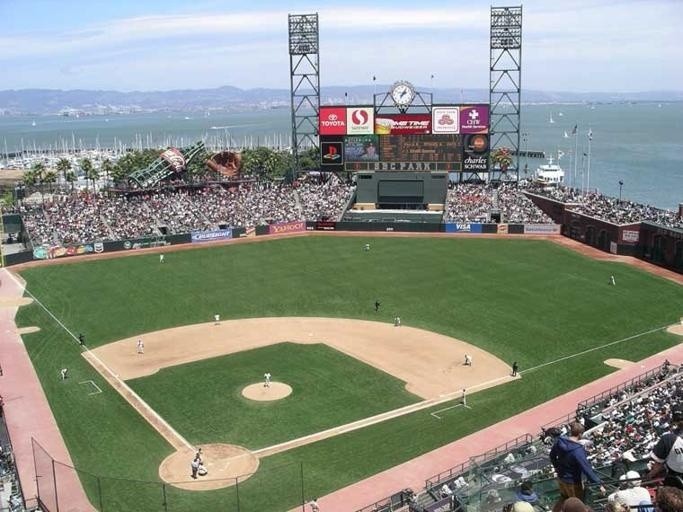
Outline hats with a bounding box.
[620,471,642,484]
[511,501,534,512]
[561,497,588,512]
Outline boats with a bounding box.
[530,152,565,187]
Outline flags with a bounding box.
[557,124,592,160]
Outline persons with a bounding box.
[79,333,86,346]
[376,299,382,312]
[607,271,616,285]
[159,253,166,263]
[394,316,400,326]
[136,339,144,354]
[214,313,220,325]
[360,142,379,161]
